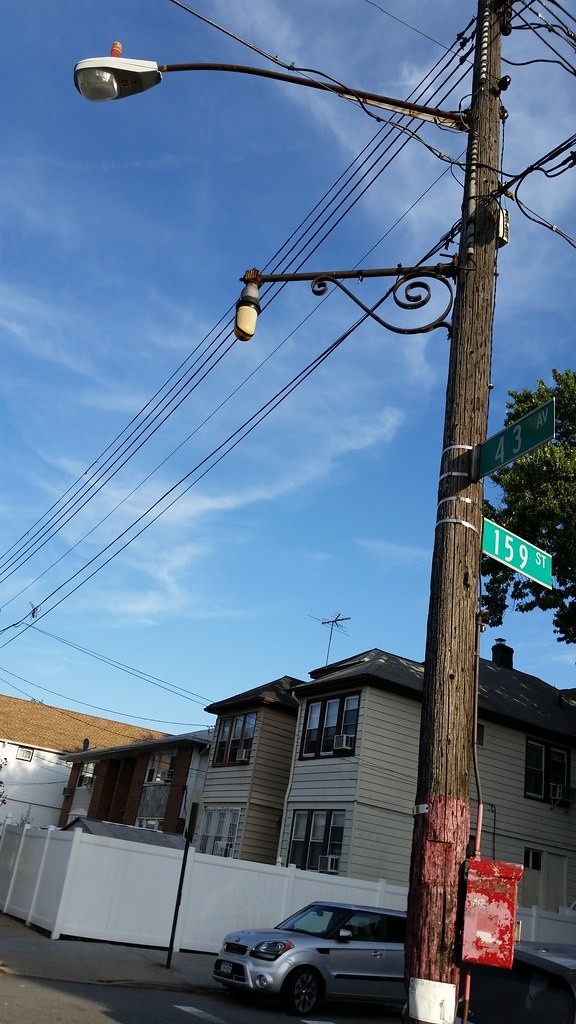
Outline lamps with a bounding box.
[318,855,340,872]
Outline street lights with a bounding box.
[72,37,507,1024]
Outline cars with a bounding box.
[211,898,411,1020]
[459,938,576,1024]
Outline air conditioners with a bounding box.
[236,749,251,760]
[549,783,576,802]
[332,734,354,749]
